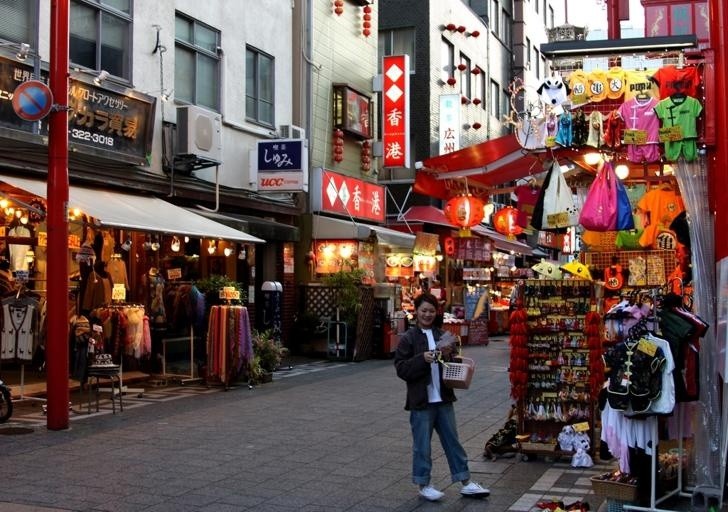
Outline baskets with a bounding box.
[605,496,632,512]
[590,473,639,502]
[442,355,475,389]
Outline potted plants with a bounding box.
[253,328,288,385]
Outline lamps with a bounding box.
[121,236,249,260]
[0,40,32,63]
[146,87,174,102]
[77,67,109,85]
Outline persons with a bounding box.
[393,293,490,501]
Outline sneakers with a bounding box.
[418,485,446,502]
[461,481,491,498]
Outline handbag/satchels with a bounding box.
[529,159,635,232]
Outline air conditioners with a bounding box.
[174,102,224,165]
[280,124,306,142]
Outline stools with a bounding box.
[76,365,124,416]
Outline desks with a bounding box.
[489,307,510,336]
[440,320,470,347]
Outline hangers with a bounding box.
[9,283,29,310]
[599,284,688,348]
